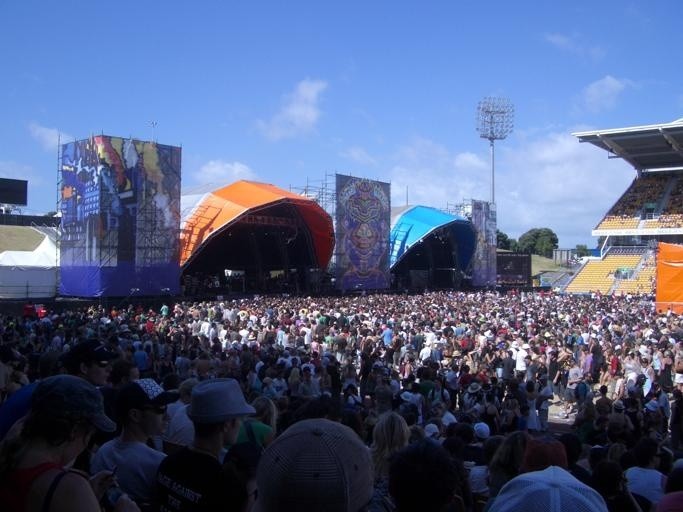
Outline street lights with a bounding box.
[475,94,516,206]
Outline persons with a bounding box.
[187,269,288,286]
[605,174,683,228]
[635,244,655,286]
[1,286,682,512]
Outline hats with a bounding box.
[34,374,116,432]
[426,424,439,436]
[186,378,256,415]
[507,350,513,355]
[525,355,531,359]
[118,378,182,410]
[600,385,607,390]
[645,400,658,409]
[401,391,412,401]
[468,382,481,393]
[540,375,547,379]
[474,423,490,438]
[613,399,625,408]
[524,440,568,469]
[639,374,648,380]
[251,418,373,512]
[70,342,119,367]
